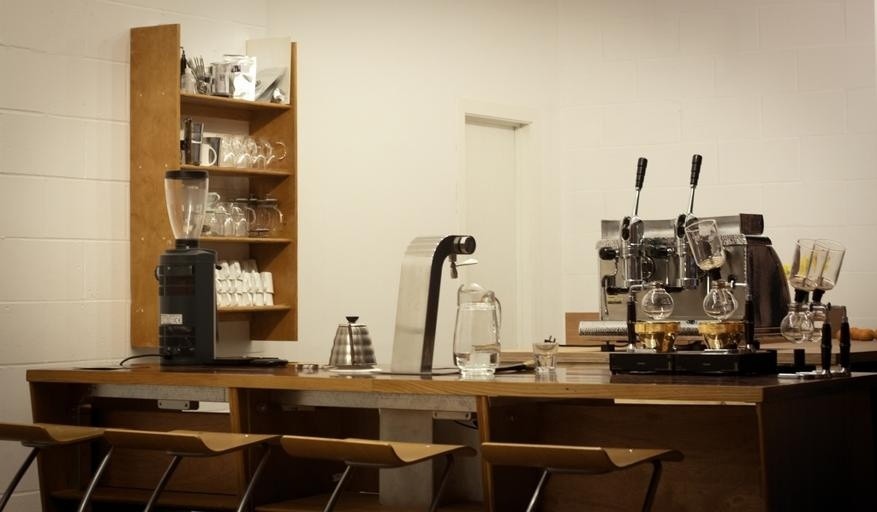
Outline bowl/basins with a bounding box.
[694,319,747,349]
[632,320,681,354]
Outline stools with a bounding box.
[104,428,283,512]
[281,436,478,512]
[2,420,114,512]
[480,441,683,512]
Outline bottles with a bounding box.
[785,236,829,292]
[805,238,847,292]
[451,281,504,376]
[682,217,726,273]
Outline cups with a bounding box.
[179,115,287,306]
[531,341,559,374]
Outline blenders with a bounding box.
[154,166,219,368]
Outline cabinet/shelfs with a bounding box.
[126,23,301,351]
